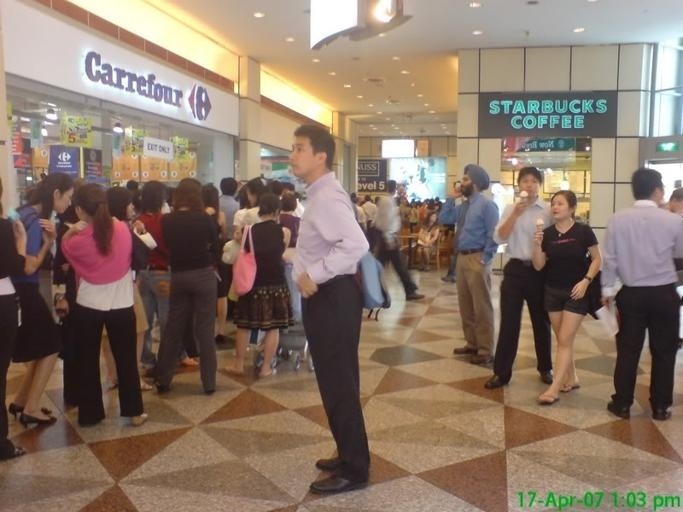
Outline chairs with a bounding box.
[440,232,453,267]
[428,234,442,269]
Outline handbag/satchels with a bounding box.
[417,232,432,247]
[566,271,607,317]
[232,248,258,297]
[222,239,242,266]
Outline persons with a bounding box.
[532,190,602,406]
[0,171,305,458]
[287,124,370,495]
[438,163,499,365]
[647,188,682,354]
[484,167,554,389]
[349,180,468,302]
[599,169,682,420]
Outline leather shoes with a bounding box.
[155,383,174,394]
[80,419,102,426]
[315,456,344,474]
[538,370,554,385]
[204,389,216,395]
[310,471,368,495]
[484,374,511,390]
[9,447,26,458]
[214,333,236,346]
[453,347,477,355]
[469,354,495,365]
[406,290,425,302]
[128,412,149,426]
[652,407,673,420]
[607,401,631,419]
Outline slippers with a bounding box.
[535,393,559,405]
[220,364,245,376]
[559,381,581,393]
[258,368,277,380]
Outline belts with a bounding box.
[510,258,537,269]
[460,246,485,254]
[315,274,355,288]
[139,264,169,271]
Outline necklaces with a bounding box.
[556,220,573,237]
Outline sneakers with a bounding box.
[440,275,456,284]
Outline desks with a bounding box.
[395,235,418,270]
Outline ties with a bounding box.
[452,201,471,252]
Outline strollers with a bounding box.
[254,263,323,373]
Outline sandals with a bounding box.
[138,361,158,372]
[107,375,154,392]
[174,358,199,373]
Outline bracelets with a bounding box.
[584,276,592,283]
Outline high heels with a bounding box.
[8,403,52,419]
[20,411,57,429]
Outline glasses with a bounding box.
[61,190,74,203]
